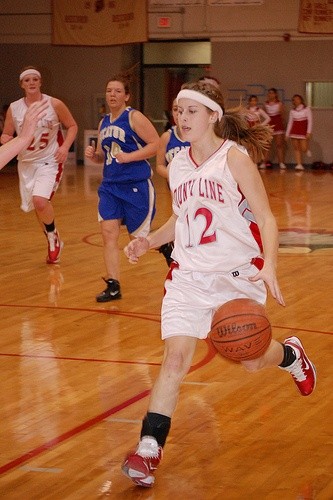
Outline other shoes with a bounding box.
[260,163,266,168]
[296,164,305,170]
[280,163,287,168]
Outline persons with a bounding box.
[154,98,191,188]
[264,88,286,169]
[200,76,219,88]
[118,80,317,488]
[85,78,174,302]
[283,93,313,169]
[0,66,77,264]
[0,98,49,169]
[244,95,271,168]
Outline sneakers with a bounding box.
[96,276,121,302]
[157,242,175,266]
[41,228,64,263]
[120,437,163,486]
[277,336,316,395]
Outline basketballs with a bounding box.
[212,299,272,361]
